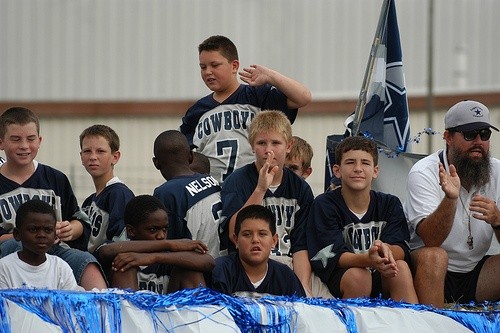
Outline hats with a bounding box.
[444,100,499,131]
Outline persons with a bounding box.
[93,195,216,290]
[0,106,110,289]
[79,125,136,252]
[0,200,82,291]
[179,35,311,183]
[190,152,210,175]
[209,205,306,297]
[153,131,227,261]
[307,136,419,304]
[404,100,500,307]
[220,110,314,296]
[285,136,313,180]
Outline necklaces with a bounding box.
[459,193,473,250]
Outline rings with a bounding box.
[445,182,447,185]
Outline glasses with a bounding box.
[454,128,493,141]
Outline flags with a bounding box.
[346,0,410,153]
